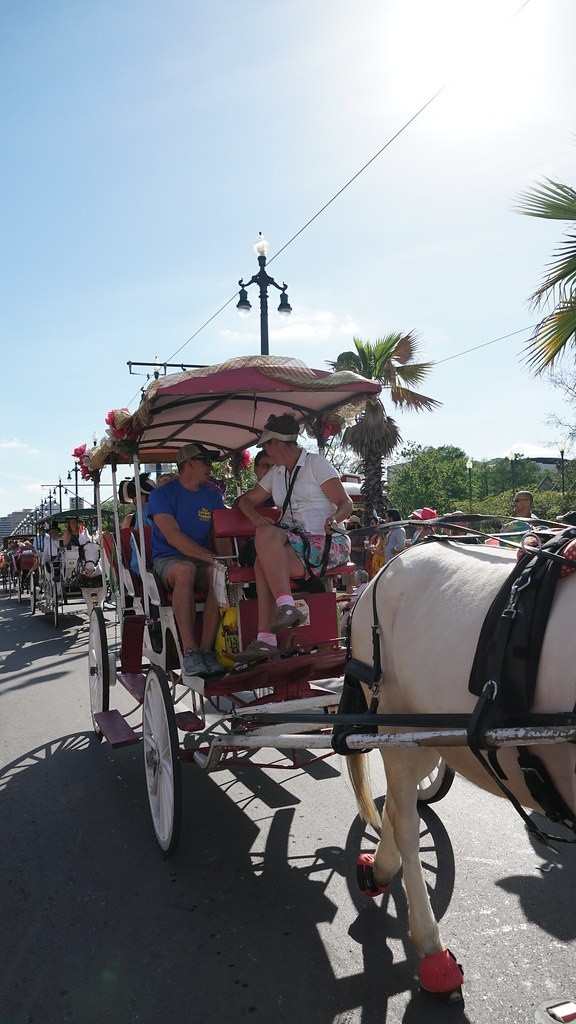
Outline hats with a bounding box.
[413,507,437,520]
[176,443,220,465]
[344,515,360,523]
[556,511,576,526]
[119,473,156,504]
[407,509,423,517]
[46,521,61,533]
[256,428,298,446]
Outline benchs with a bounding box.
[12,507,357,607]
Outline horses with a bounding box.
[78,523,108,616]
[341,531,576,991]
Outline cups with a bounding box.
[369,545,375,554]
[405,539,411,548]
[364,541,369,549]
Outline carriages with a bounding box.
[78,352,575,994]
[0,508,118,630]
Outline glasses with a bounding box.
[258,463,273,468]
[191,457,211,465]
[513,498,528,502]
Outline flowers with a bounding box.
[299,411,347,450]
[220,449,253,481]
[70,408,138,482]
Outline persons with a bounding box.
[0,538,34,564]
[337,569,369,636]
[498,490,576,546]
[121,473,227,636]
[232,449,276,601]
[147,444,226,676]
[233,415,354,662]
[33,522,61,573]
[63,516,81,550]
[346,507,477,579]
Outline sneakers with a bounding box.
[180,651,208,676]
[232,641,278,662]
[270,604,307,634]
[201,649,226,675]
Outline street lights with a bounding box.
[464,458,474,514]
[235,228,293,356]
[11,354,161,535]
[507,451,515,494]
[558,439,566,495]
[482,456,490,496]
